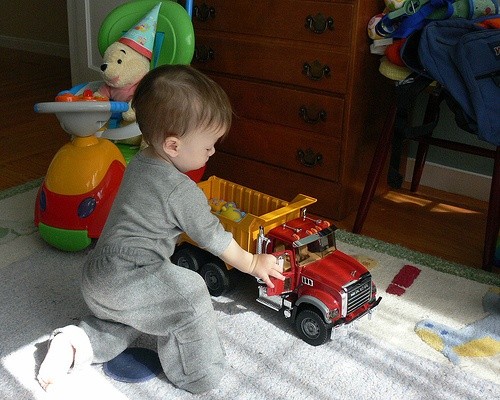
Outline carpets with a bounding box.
[0,176,500,400]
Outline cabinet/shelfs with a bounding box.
[176,0,416,221]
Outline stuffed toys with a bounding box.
[95,2,163,123]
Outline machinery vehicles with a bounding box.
[172,174,383,346]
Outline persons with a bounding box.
[37,63,286,395]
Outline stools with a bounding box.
[351,56,500,272]
